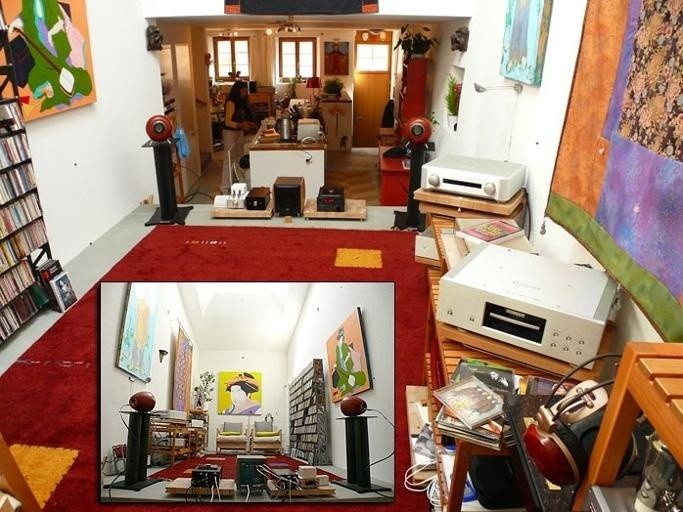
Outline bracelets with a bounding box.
[232,122,238,129]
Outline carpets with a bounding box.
[0,226,444,511]
[148,454,346,481]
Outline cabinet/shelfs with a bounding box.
[0,1,58,349]
[423,213,683,512]
[289,358,333,466]
[147,410,209,466]
[318,91,352,152]
[398,58,428,129]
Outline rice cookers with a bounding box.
[297,117,322,143]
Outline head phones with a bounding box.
[523,353,640,488]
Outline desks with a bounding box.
[248,86,275,117]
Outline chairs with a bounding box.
[216,422,283,457]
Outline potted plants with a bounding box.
[445,71,460,134]
[323,79,345,100]
[193,371,215,411]
[394,24,441,59]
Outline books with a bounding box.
[433,355,576,452]
[1,103,79,346]
[455,220,526,245]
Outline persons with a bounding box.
[219,81,257,195]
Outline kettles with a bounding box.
[276,116,292,142]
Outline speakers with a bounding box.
[340,395,367,416]
[400,117,433,145]
[129,391,156,412]
[145,115,172,142]
